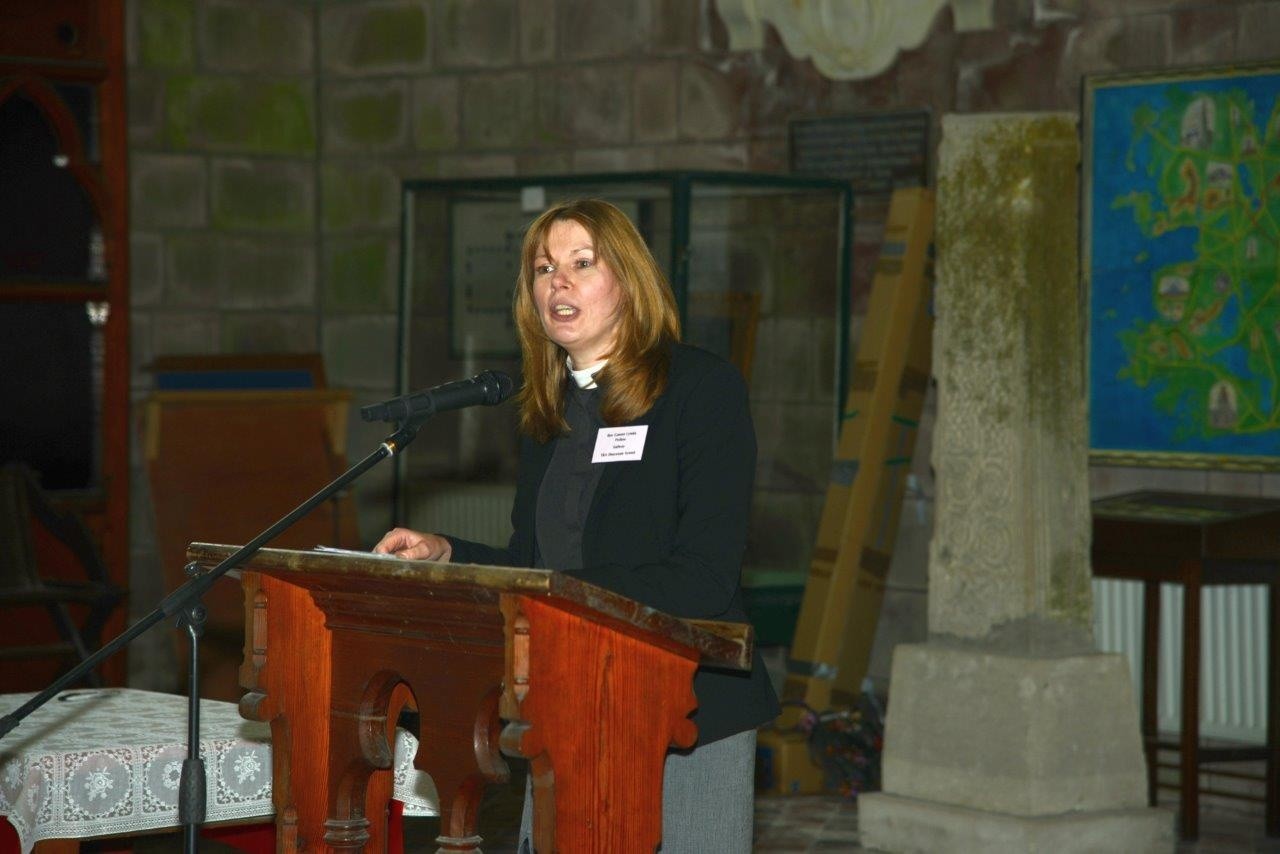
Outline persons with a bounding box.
[373,196,783,854]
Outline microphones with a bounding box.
[360,370,514,421]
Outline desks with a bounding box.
[1092,485,1280,845]
[0,684,440,854]
